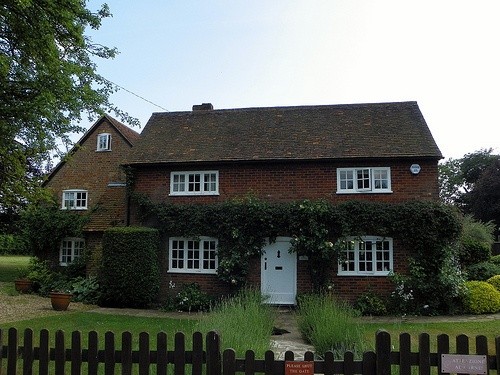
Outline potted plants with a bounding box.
[49,286,73,311]
[14,267,34,294]
